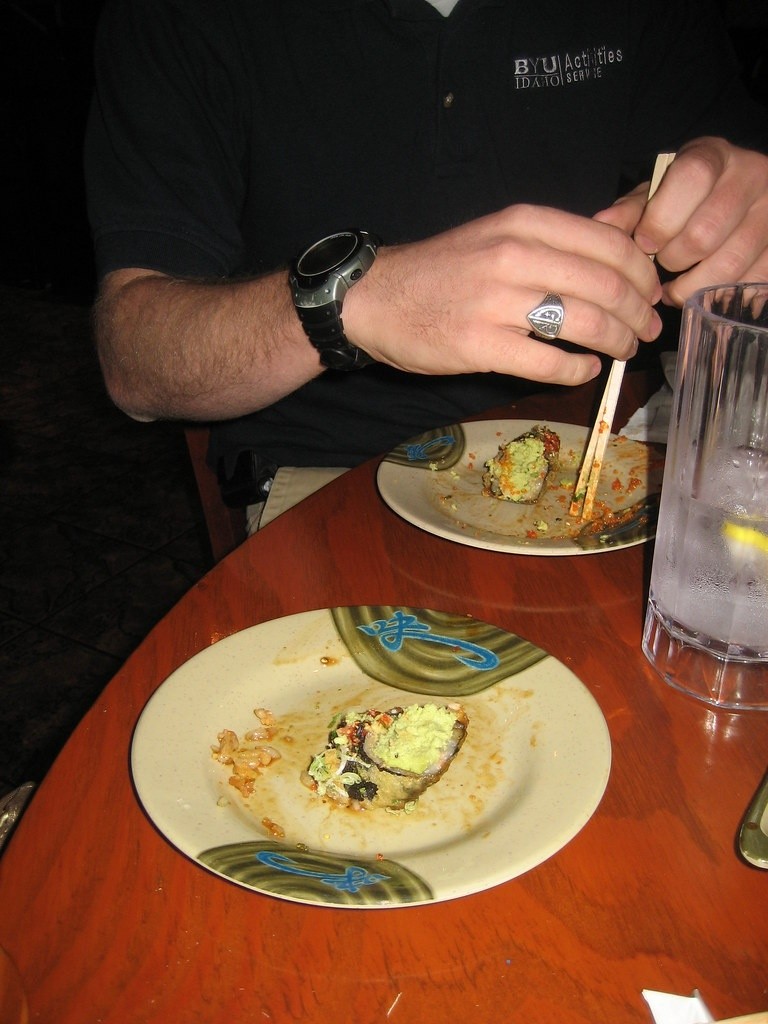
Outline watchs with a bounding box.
[288,228,386,370]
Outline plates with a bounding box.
[375,417,661,557]
[127,603,614,912]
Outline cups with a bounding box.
[638,280,768,712]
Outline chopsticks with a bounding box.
[565,150,678,523]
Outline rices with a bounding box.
[211,707,318,842]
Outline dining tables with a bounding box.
[0,359,768,1024]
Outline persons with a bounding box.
[84,0,768,538]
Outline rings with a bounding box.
[528,292,564,340]
